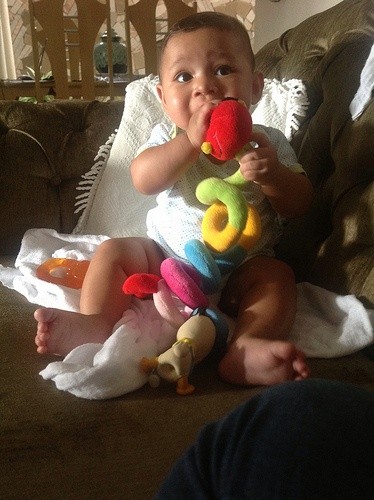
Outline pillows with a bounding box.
[72,73,310,238]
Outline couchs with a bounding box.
[0,1,374,500]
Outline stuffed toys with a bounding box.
[121,97,267,395]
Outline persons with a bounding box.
[31,11,316,390]
